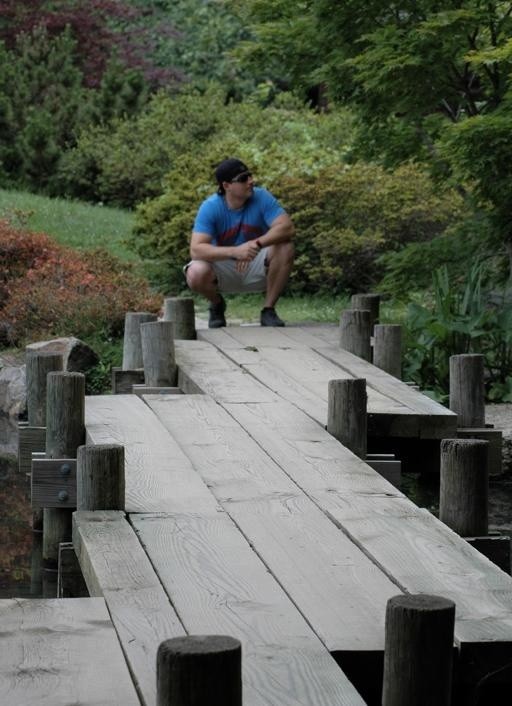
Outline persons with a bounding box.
[182,156,297,329]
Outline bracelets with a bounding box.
[255,238,263,249]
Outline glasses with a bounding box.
[232,172,252,183]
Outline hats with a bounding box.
[215,158,249,189]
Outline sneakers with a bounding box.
[208,293,226,328]
[260,307,285,326]
